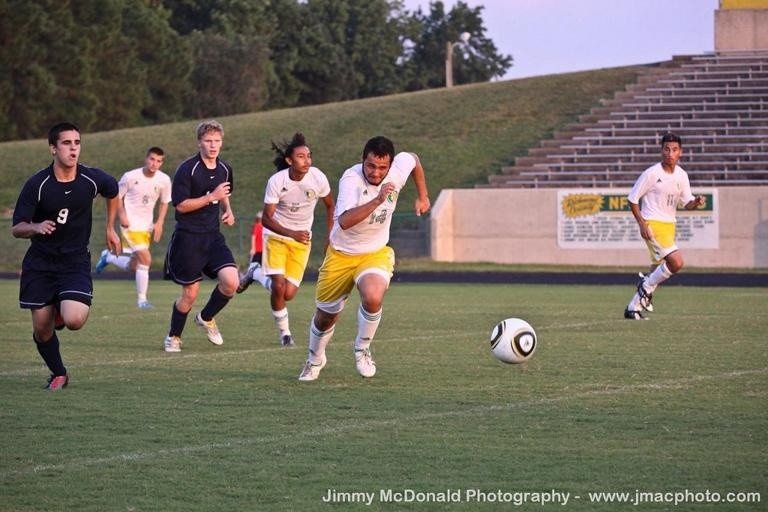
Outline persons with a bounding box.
[624,133,707,322]
[299,136,430,381]
[95,148,173,309]
[249,209,265,265]
[165,120,242,353]
[12,122,116,390]
[236,133,333,342]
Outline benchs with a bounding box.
[506,48,768,186]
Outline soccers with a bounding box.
[490,317,538,364]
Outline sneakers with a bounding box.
[624,305,650,320]
[44,369,69,391]
[354,341,376,377]
[194,310,224,346]
[638,281,654,312]
[54,310,67,331]
[95,248,111,274]
[237,261,260,294]
[163,334,182,354]
[137,299,154,309]
[298,352,327,382]
[280,334,296,348]
